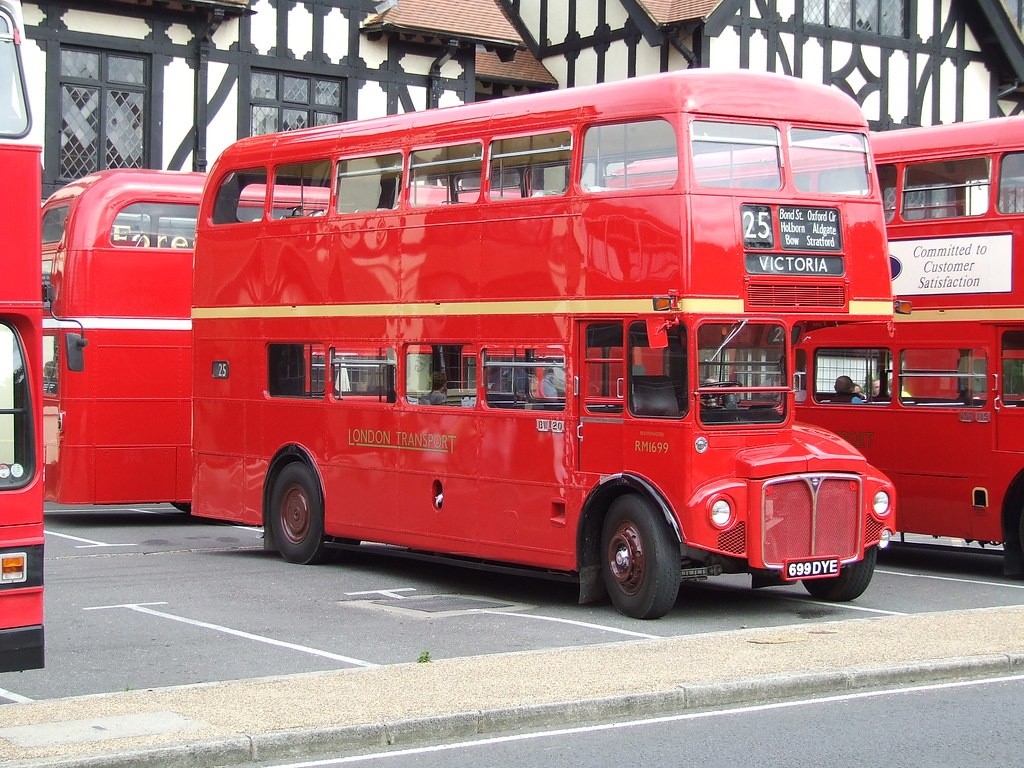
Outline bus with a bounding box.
[0,0,44,673]
[191,68,897,621]
[43,169,564,526]
[606,113,1024,574]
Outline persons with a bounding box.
[425,370,448,406]
[829,376,912,404]
[535,368,557,403]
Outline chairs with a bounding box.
[814,392,861,403]
[630,375,679,417]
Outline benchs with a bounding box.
[486,400,624,413]
